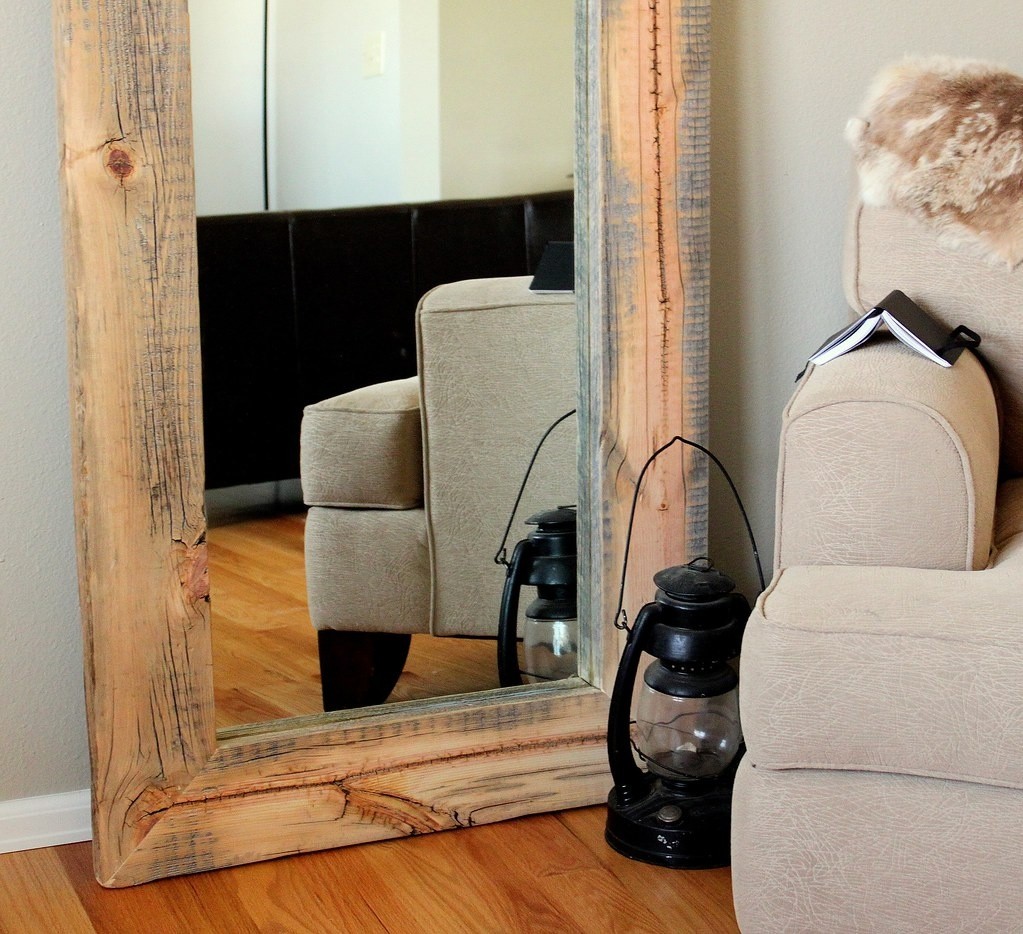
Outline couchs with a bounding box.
[731,193,1023,934]
[291,272,578,712]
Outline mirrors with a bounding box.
[50,0,714,892]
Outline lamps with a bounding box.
[493,407,578,689]
[603,434,767,871]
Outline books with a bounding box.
[794,292,981,385]
[528,239,576,294]
